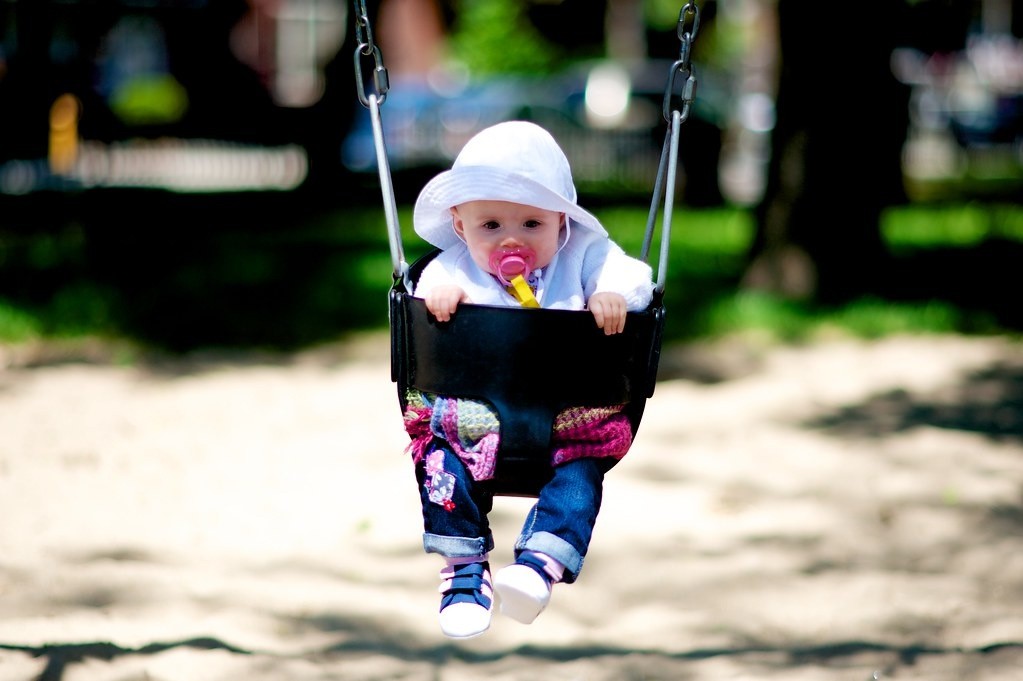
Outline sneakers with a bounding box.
[494,555,566,624]
[437,557,498,639]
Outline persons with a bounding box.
[411,121,653,640]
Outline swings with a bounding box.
[351,1,703,505]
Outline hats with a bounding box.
[412,120,610,251]
